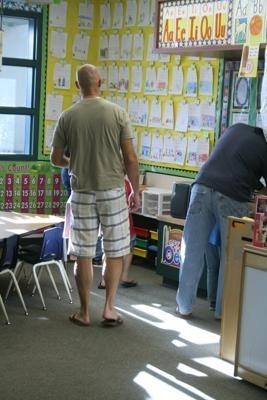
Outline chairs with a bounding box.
[0,211,75,325]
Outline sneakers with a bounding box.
[93,259,103,267]
[208,301,216,310]
[175,304,192,316]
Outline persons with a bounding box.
[49,63,142,327]
[204,222,220,312]
[99,150,138,290]
[175,122,266,317]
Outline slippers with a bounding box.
[101,314,123,327]
[120,280,137,287]
[98,282,107,289]
[69,312,90,326]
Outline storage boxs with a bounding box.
[135,186,172,218]
[131,227,158,259]
